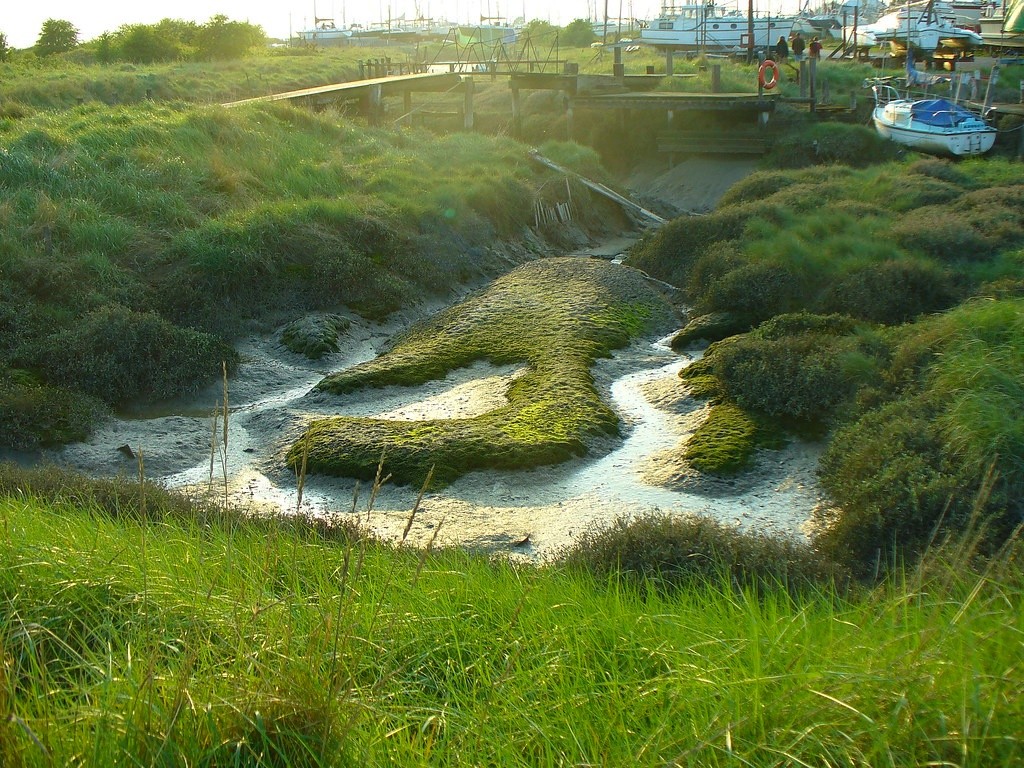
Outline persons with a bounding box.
[792,32,805,62]
[809,36,822,62]
[775,36,789,64]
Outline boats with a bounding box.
[640,5,794,53]
[842,0,983,49]
[297,18,351,38]
[806,0,867,29]
[873,85,998,158]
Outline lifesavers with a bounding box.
[758,60,779,89]
